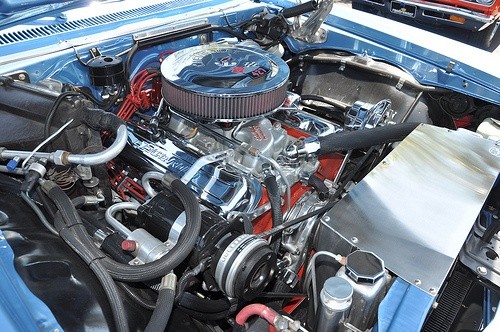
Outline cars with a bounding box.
[350,0,500,50]
